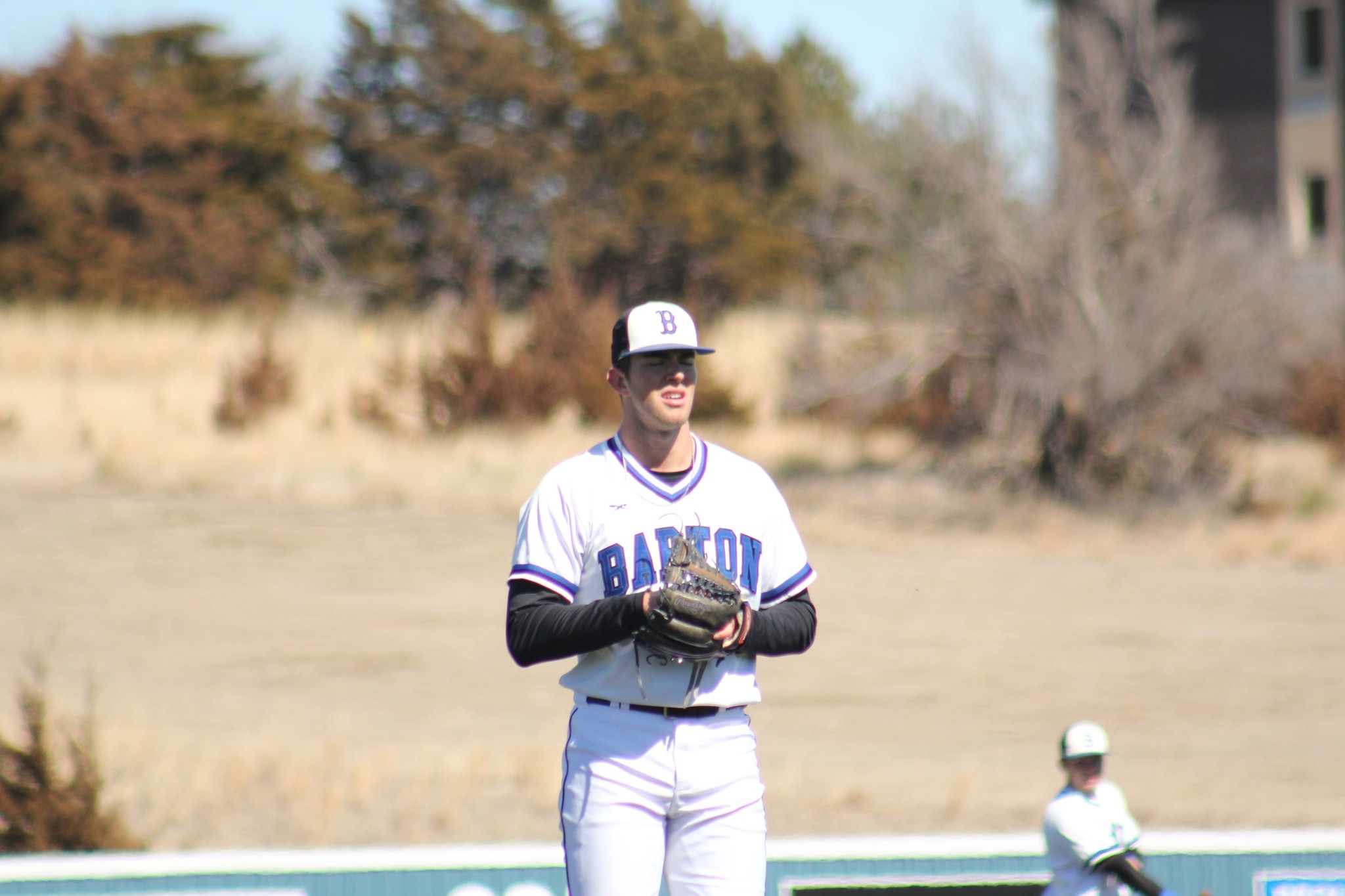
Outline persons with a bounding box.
[1044,721,1176,896]
[505,300,816,896]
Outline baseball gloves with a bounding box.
[631,533,742,663]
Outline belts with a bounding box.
[586,696,746,717]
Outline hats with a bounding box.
[611,301,715,363]
[1062,723,1109,758]
